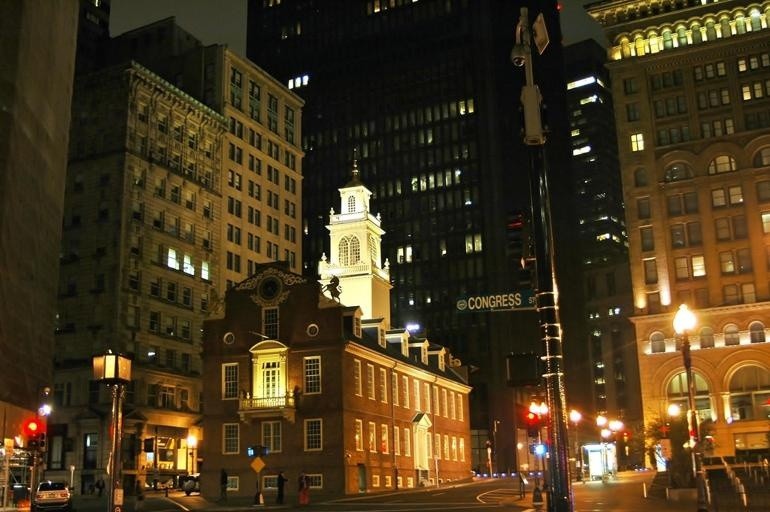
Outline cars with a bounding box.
[33,480,70,510]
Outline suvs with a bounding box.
[179,473,199,496]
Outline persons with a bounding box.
[276,471,288,504]
[95,477,105,498]
[298,471,311,504]
[219,469,228,501]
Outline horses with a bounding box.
[322,276,343,305]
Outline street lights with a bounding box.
[596,415,624,473]
[91,351,132,512]
[568,409,584,482]
[530,395,549,490]
[672,303,708,511]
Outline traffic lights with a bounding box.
[523,409,539,438]
[22,415,46,449]
